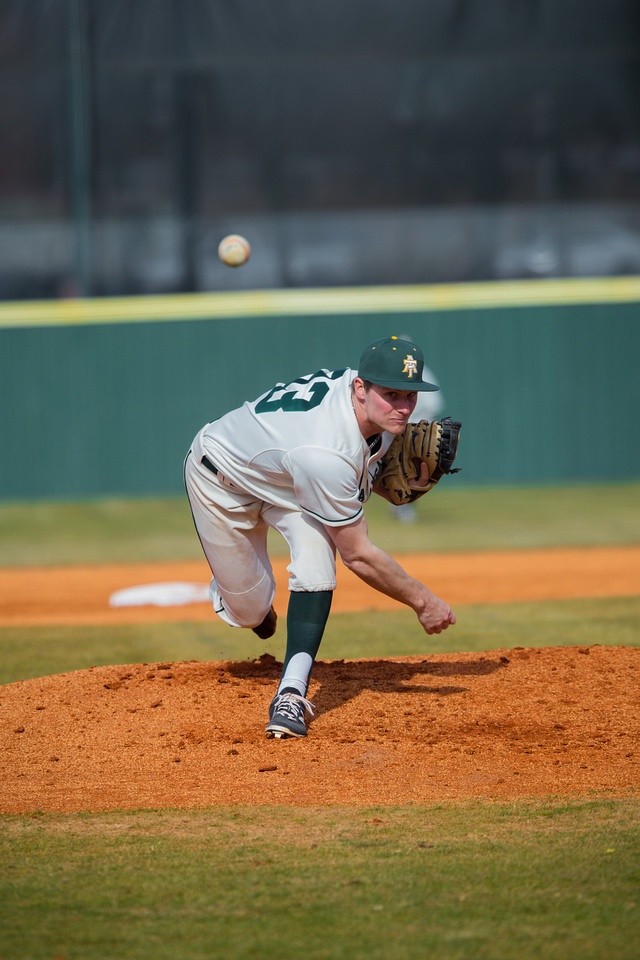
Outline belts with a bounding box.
[201,456,233,486]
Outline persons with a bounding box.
[183,336,455,737]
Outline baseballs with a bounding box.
[216,235,250,268]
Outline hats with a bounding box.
[358,336,439,392]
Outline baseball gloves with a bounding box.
[371,416,463,505]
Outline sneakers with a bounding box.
[252,604,277,639]
[266,687,315,737]
